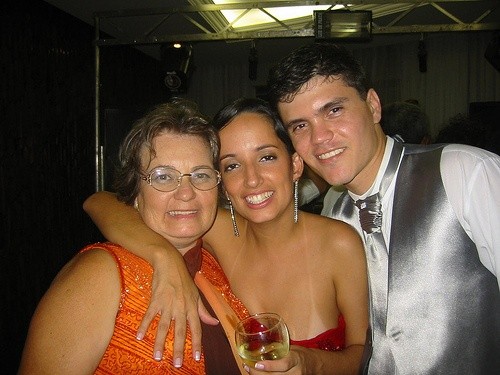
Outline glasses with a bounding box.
[140,165,222,192]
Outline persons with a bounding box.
[22,94,220,374]
[268,43,500,375]
[81,93,370,374]
[269,101,500,217]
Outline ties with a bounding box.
[349,136,403,337]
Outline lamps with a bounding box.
[314,3,372,41]
[164,45,193,89]
[248,47,257,80]
[417,41,427,72]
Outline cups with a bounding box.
[236,312,290,368]
